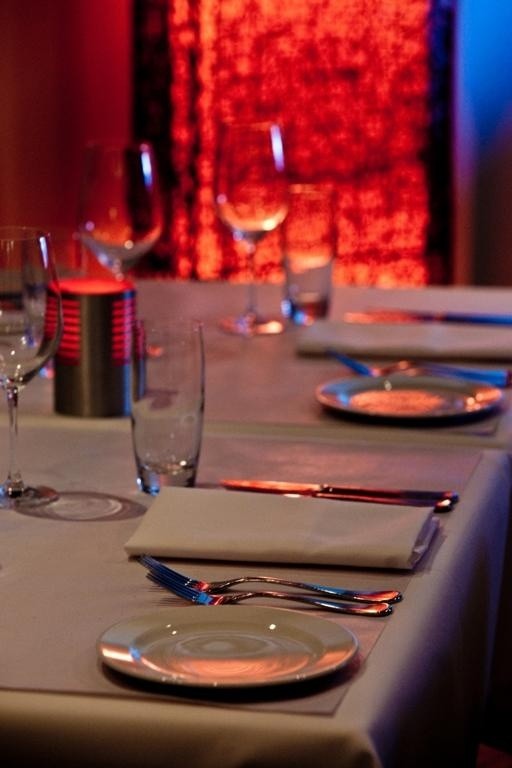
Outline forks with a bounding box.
[143,568,395,620]
[133,548,406,602]
[320,342,508,383]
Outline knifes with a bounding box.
[221,477,460,512]
[347,308,511,330]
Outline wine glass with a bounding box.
[217,118,290,337]
[0,224,62,505]
[76,141,166,281]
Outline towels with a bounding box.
[123,485,439,573]
[294,320,512,361]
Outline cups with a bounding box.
[130,317,202,494]
[288,240,334,323]
[54,279,135,416]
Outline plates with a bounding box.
[96,601,359,697]
[312,374,504,426]
